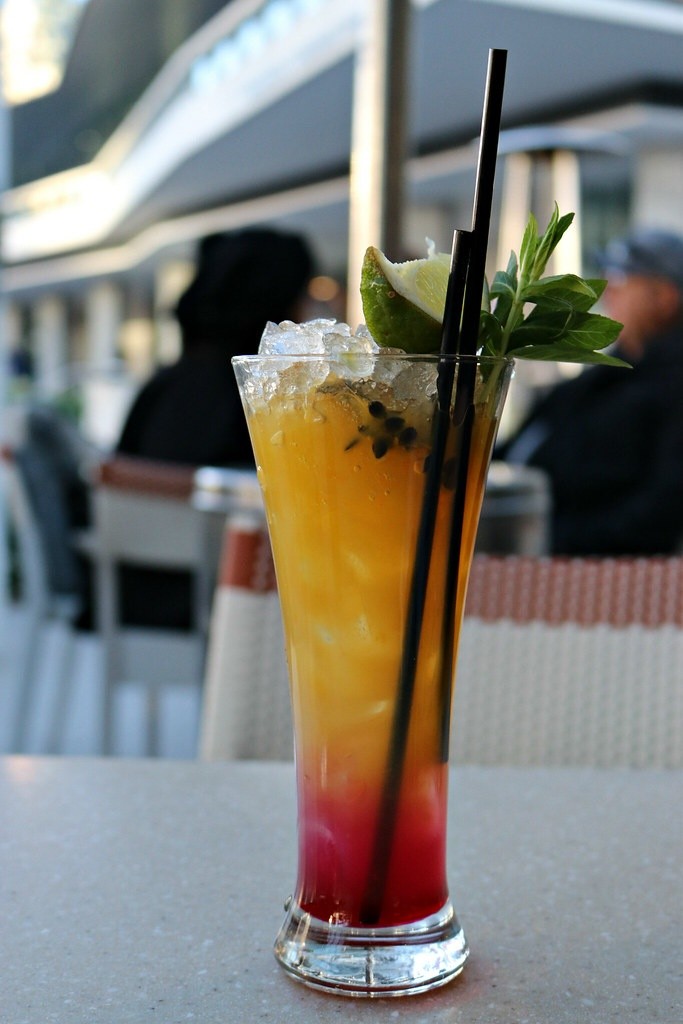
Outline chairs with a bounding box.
[1,404,103,757]
[94,460,229,754]
[198,514,682,771]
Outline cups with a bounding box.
[232,353,512,998]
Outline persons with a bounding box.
[66,219,318,629]
[494,229,682,559]
